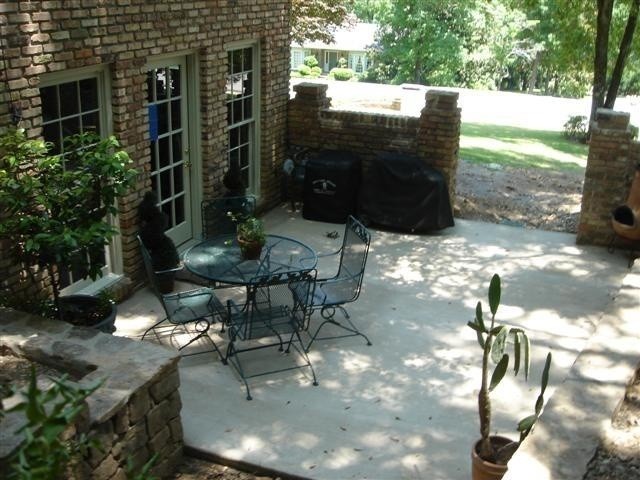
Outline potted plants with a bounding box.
[466,273,552,480]
[0,127,144,336]
